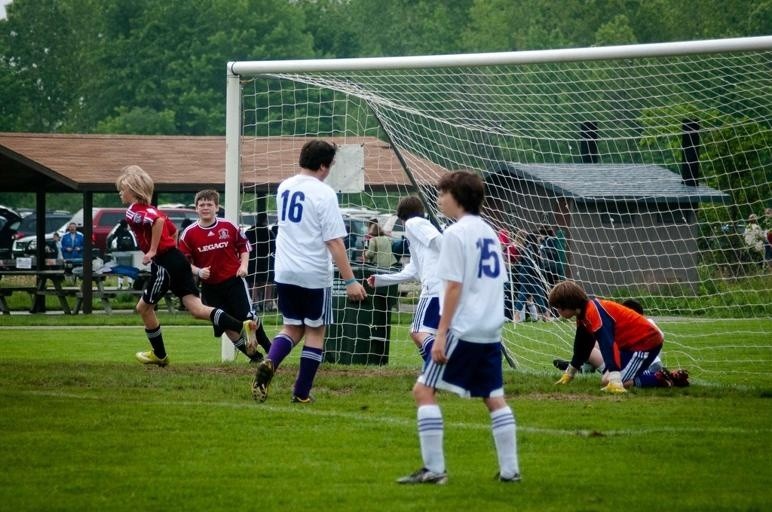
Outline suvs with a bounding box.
[74,206,203,261]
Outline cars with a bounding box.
[0,206,76,248]
[339,211,412,260]
[11,211,85,270]
[239,210,278,236]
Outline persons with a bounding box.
[252,140,368,402]
[552,299,663,375]
[53,219,200,312]
[396,172,523,485]
[494,224,564,323]
[744,208,772,267]
[549,281,689,393]
[244,213,399,311]
[366,196,444,362]
[179,190,272,364]
[117,166,258,367]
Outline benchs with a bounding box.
[1,270,71,315]
[71,270,177,315]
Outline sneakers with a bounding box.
[240,320,274,404]
[292,395,316,405]
[491,471,522,484]
[654,369,689,387]
[397,468,448,485]
[136,351,169,366]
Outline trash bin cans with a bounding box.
[325,265,399,366]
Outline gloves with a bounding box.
[555,363,578,384]
[601,371,628,393]
